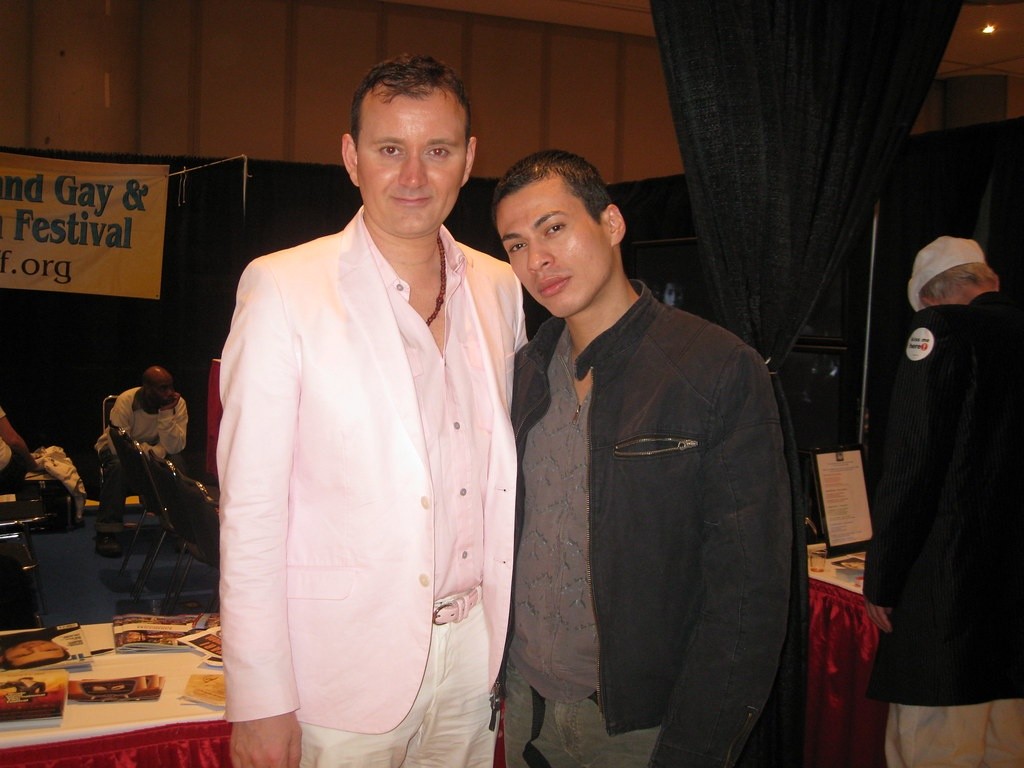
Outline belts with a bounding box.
[433,589,478,625]
[522,686,598,768]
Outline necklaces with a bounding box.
[426,238,446,325]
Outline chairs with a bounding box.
[0,480,46,629]
[100,395,221,615]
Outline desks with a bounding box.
[808,543,888,768]
[0,624,232,768]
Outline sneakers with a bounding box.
[95,532,123,556]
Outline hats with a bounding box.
[907,235,986,312]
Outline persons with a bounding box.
[92,366,188,556]
[0,406,46,492]
[212,48,528,768]
[489,148,793,768]
[862,237,1024,767]
